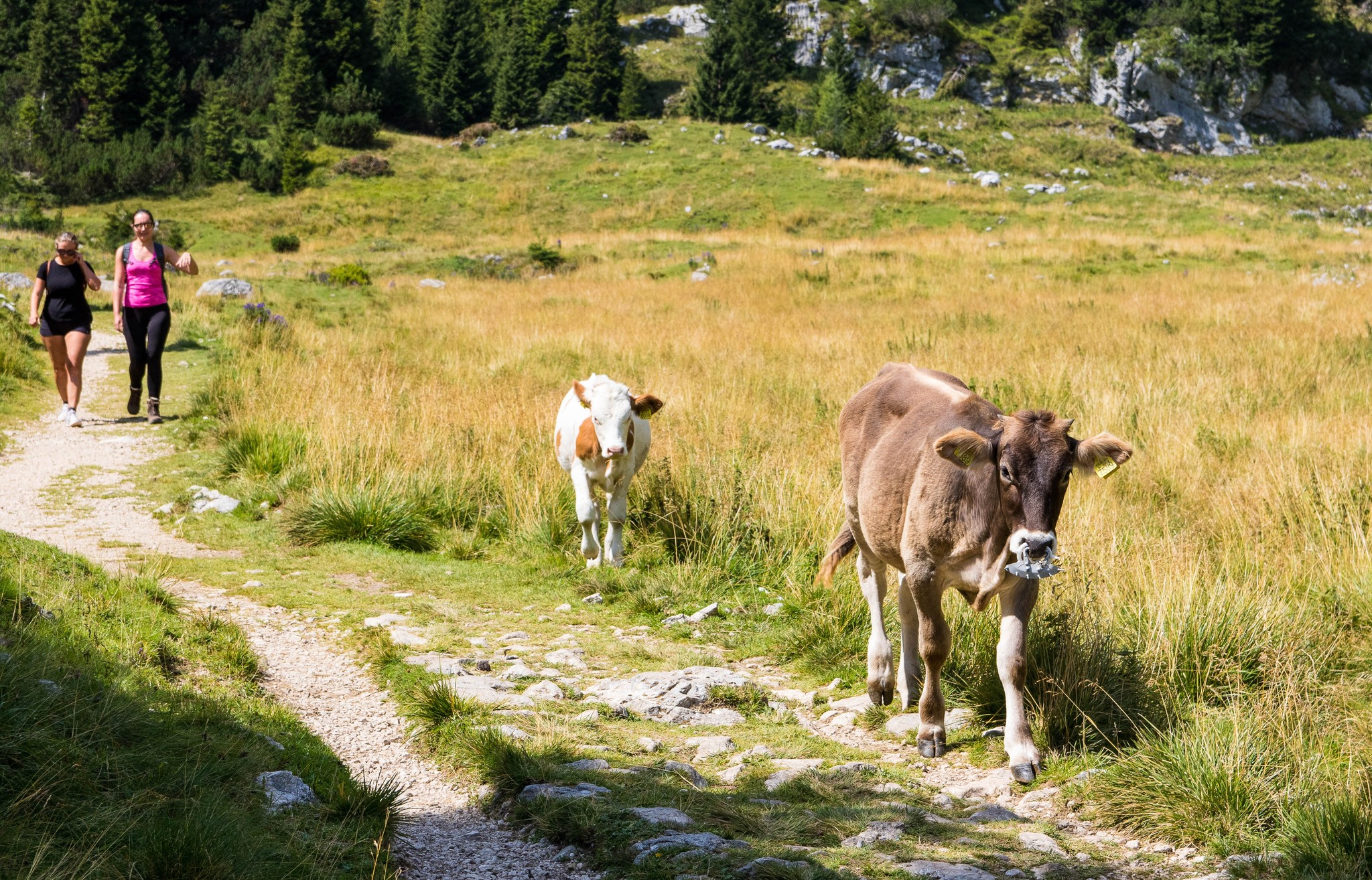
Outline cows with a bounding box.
[551,369,666,573]
[809,357,1138,789]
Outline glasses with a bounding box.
[56,244,76,256]
[134,222,153,230]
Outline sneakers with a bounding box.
[146,398,162,424]
[66,408,83,428]
[57,403,68,422]
[127,384,143,415]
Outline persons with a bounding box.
[28,232,102,426]
[112,209,199,425]
